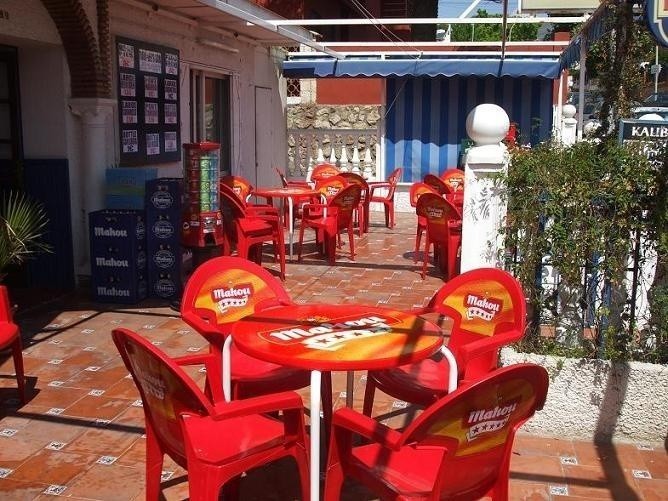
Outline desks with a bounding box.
[234,303,443,500]
[251,185,330,260]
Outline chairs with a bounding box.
[220,182,279,267]
[416,193,462,282]
[334,173,370,234]
[219,192,287,282]
[439,168,464,194]
[0,284,30,404]
[222,177,274,220]
[274,167,312,230]
[410,181,441,265]
[180,257,309,435]
[311,164,339,189]
[361,269,525,444]
[296,184,362,266]
[424,173,453,202]
[321,177,347,247]
[369,167,402,229]
[322,362,549,501]
[112,327,308,501]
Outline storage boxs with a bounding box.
[88,245,149,275]
[148,273,190,302]
[148,246,190,273]
[89,275,148,302]
[105,168,158,210]
[88,212,147,244]
[147,177,182,212]
[147,214,182,245]
